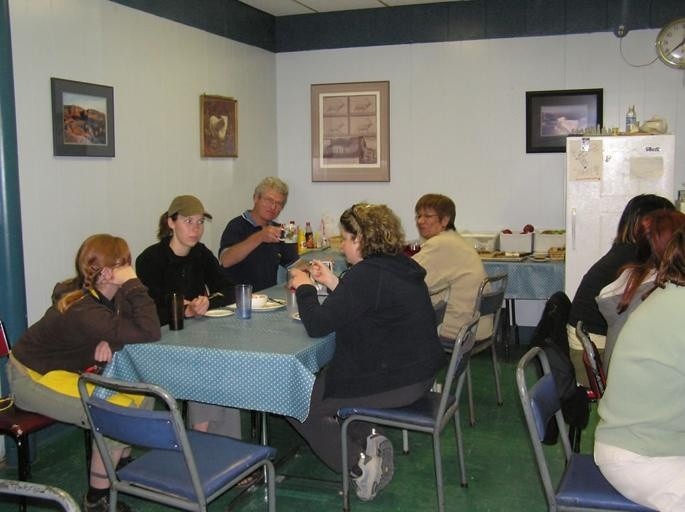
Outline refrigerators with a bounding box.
[565,134,674,302]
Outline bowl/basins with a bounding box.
[533,252,548,259]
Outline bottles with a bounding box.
[625,106,637,133]
[291,269,311,322]
[289,221,314,255]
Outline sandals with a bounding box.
[236,475,257,488]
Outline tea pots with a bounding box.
[639,116,667,134]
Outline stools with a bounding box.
[0,321,57,512]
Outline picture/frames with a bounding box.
[50,77,115,157]
[310,80,390,182]
[526,88,603,153]
[200,95,239,158]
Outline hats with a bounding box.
[168,196,212,219]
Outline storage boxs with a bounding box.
[499,231,534,253]
[534,230,565,254]
[459,232,498,252]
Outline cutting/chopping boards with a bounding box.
[480,255,528,263]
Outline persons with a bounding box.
[136,195,237,326]
[565,194,685,512]
[408,194,495,392]
[6,232,161,512]
[218,178,300,293]
[285,202,448,504]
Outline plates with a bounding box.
[530,258,550,263]
[203,309,234,317]
[227,298,287,312]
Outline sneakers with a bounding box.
[365,428,394,490]
[82,493,130,512]
[350,452,383,502]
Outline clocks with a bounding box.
[656,18,685,69]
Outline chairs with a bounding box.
[337,311,482,512]
[516,346,661,512]
[77,373,278,512]
[529,291,595,466]
[440,273,508,427]
[576,321,606,405]
[428,283,451,392]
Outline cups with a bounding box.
[234,284,253,319]
[277,224,298,243]
[253,294,268,307]
[167,291,185,331]
[411,240,421,255]
